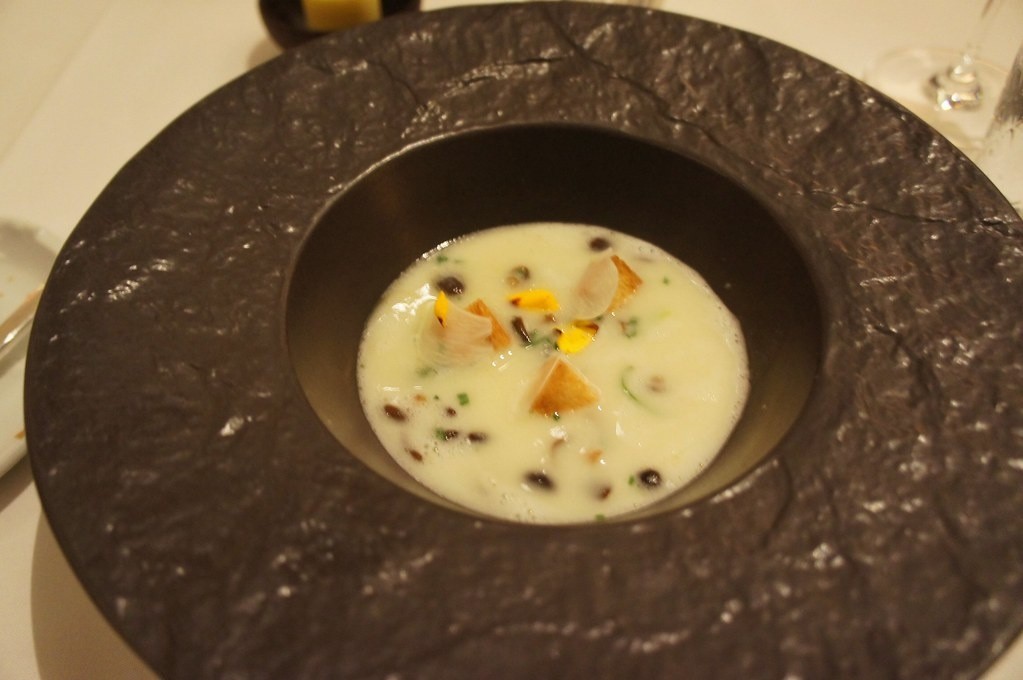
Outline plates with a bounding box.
[22,2,1021,680]
[2,217,59,475]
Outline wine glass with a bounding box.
[871,1,1007,152]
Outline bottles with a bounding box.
[253,0,420,47]
